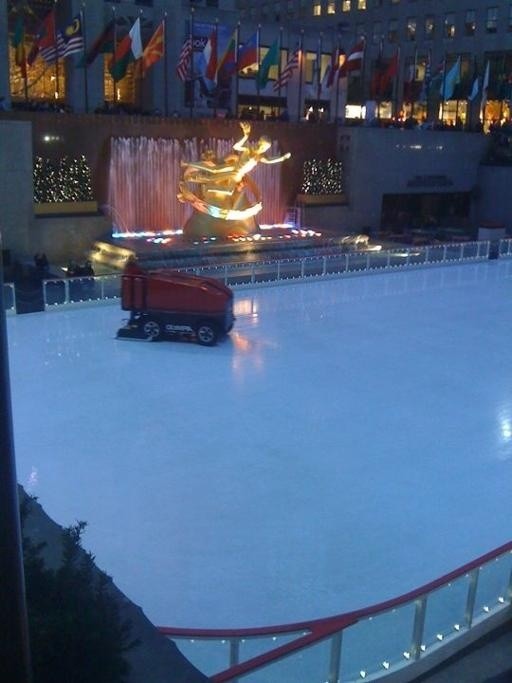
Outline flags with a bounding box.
[12,0,500,111]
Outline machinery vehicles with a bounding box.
[108,253,239,349]
